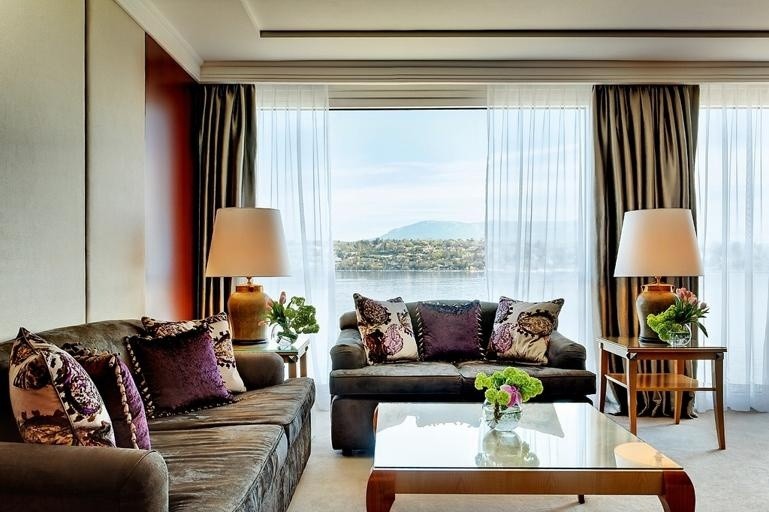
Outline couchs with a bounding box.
[0,319,315,512]
[329,299,596,456]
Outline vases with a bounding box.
[274,322,297,348]
[481,397,522,432]
[667,322,692,347]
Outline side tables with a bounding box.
[598,335,728,449]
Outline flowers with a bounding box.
[647,288,711,341]
[473,367,544,405]
[258,289,320,339]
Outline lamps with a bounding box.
[204,207,293,346]
[612,208,706,342]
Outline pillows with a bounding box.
[8,327,117,449]
[141,311,247,393]
[352,292,420,366]
[60,342,151,452]
[485,295,565,365]
[416,300,488,362]
[124,322,237,420]
